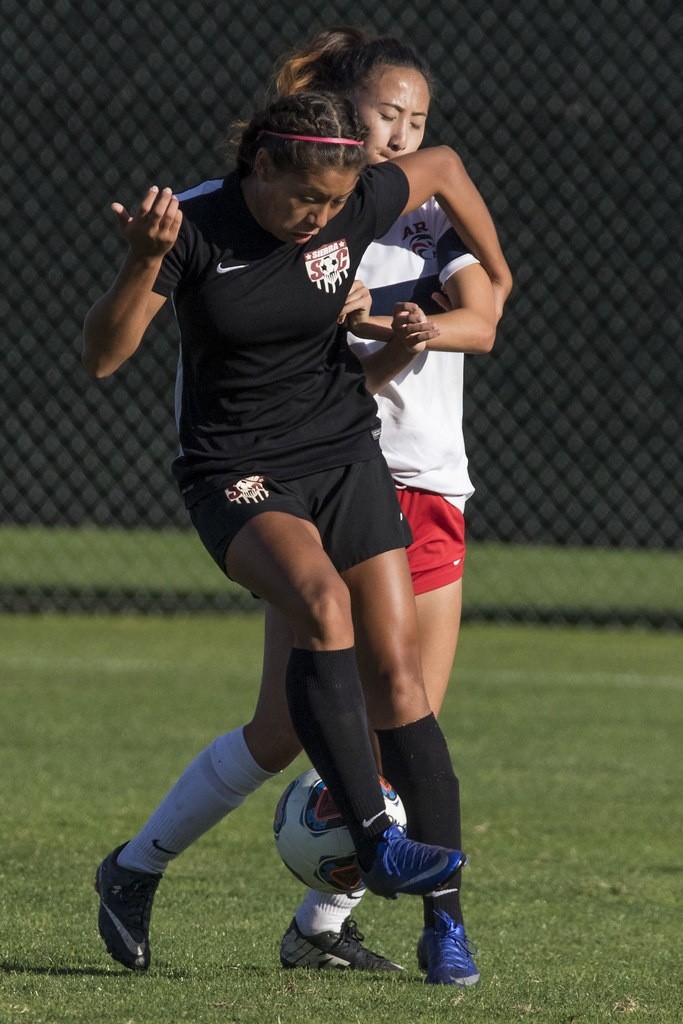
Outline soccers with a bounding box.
[272,763,407,897]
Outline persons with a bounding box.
[79,92,514,988]
[95,26,498,976]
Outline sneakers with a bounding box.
[93,840,163,970]
[279,918,407,975]
[417,908,482,987]
[360,823,465,900]
